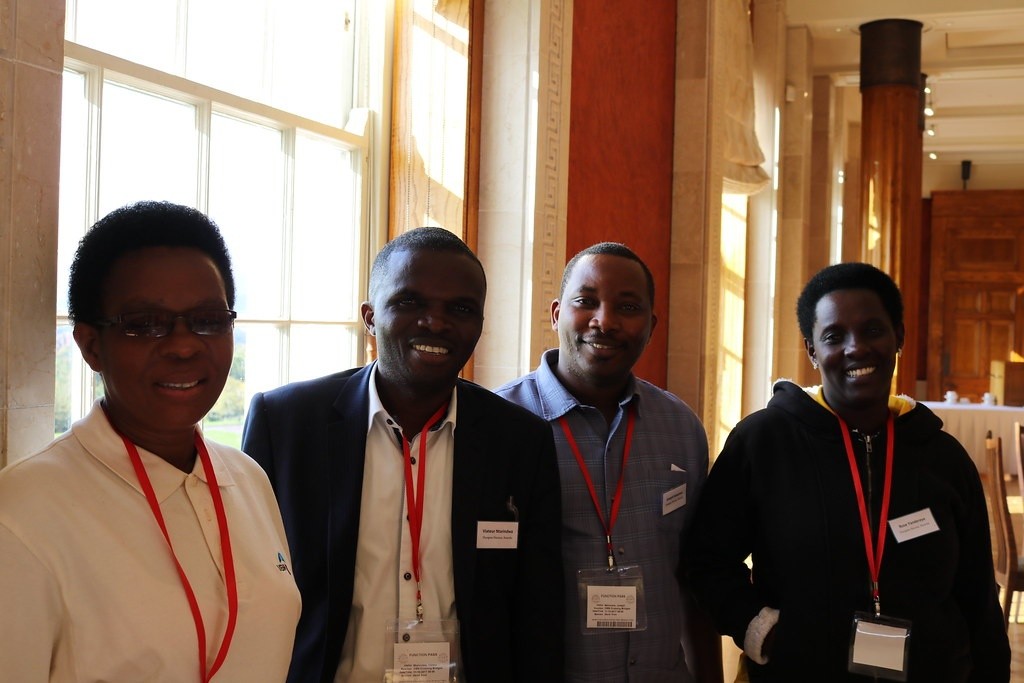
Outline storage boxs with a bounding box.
[989,359,1024,406]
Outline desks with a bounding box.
[918,401,1024,480]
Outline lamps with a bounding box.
[924,80,938,160]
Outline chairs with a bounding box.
[983,422,1024,636]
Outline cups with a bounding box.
[944,390,958,405]
[983,392,996,406]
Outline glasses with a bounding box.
[98,309,237,338]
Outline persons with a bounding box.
[0,201,301,683]
[489,243,724,683]
[239,226,566,683]
[677,262,1012,683]
